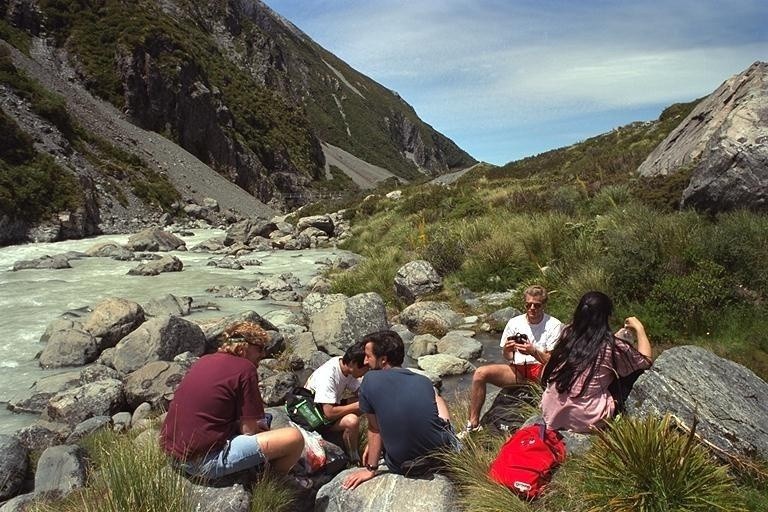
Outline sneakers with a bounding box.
[455,420,484,444]
[290,473,313,492]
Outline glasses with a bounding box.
[525,301,543,309]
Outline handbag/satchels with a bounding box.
[285,387,342,432]
[488,422,566,501]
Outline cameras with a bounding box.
[507,333,528,345]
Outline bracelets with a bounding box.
[365,462,377,472]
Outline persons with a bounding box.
[457,284,565,440]
[340,331,461,490]
[303,340,370,467]
[158,321,313,488]
[538,291,654,434]
